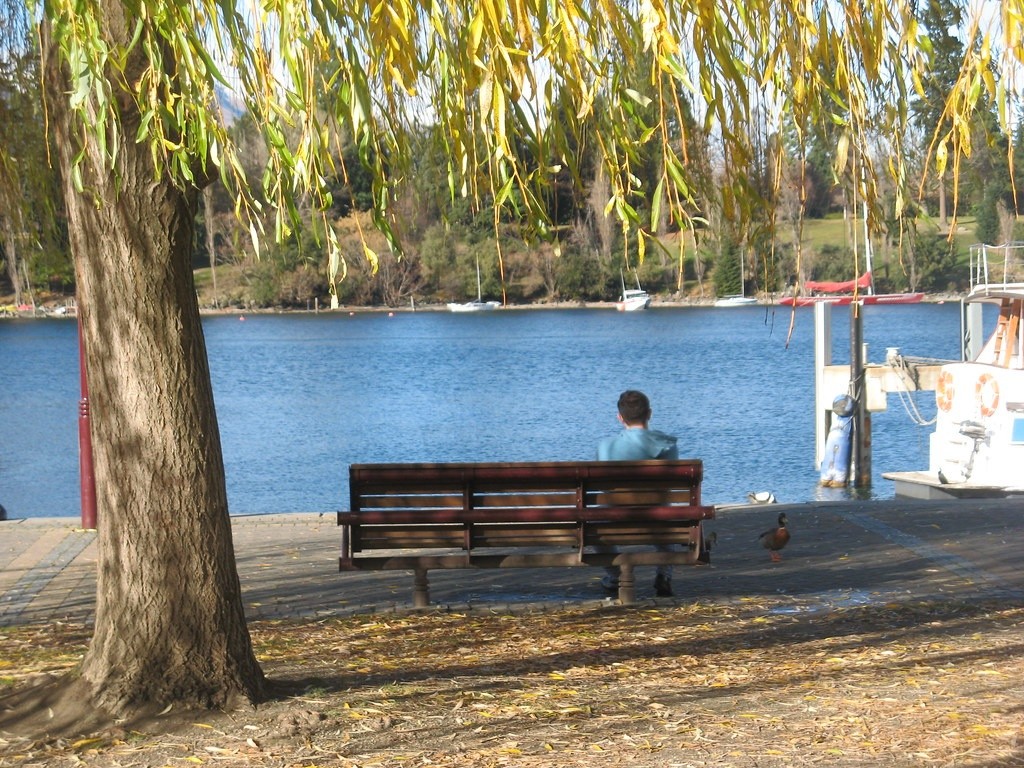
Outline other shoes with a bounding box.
[601,576,617,591]
[653,574,672,596]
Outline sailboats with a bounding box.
[713,247,760,309]
[780,156,929,303]
[446,252,502,312]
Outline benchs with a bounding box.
[336,458,715,607]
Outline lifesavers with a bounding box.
[974,373,1000,418]
[936,371,954,412]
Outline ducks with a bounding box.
[680,531,717,563]
[758,512,791,560]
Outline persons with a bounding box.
[584,390,679,597]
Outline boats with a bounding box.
[813,242,1024,501]
[615,266,651,313]
